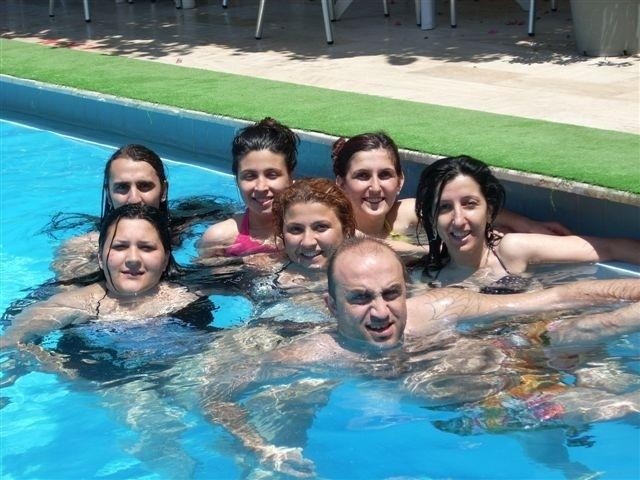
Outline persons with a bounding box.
[194,116,299,256]
[52,144,199,280]
[332,132,569,248]
[169,236,640,479]
[1,200,214,383]
[196,177,357,305]
[410,154,640,295]
